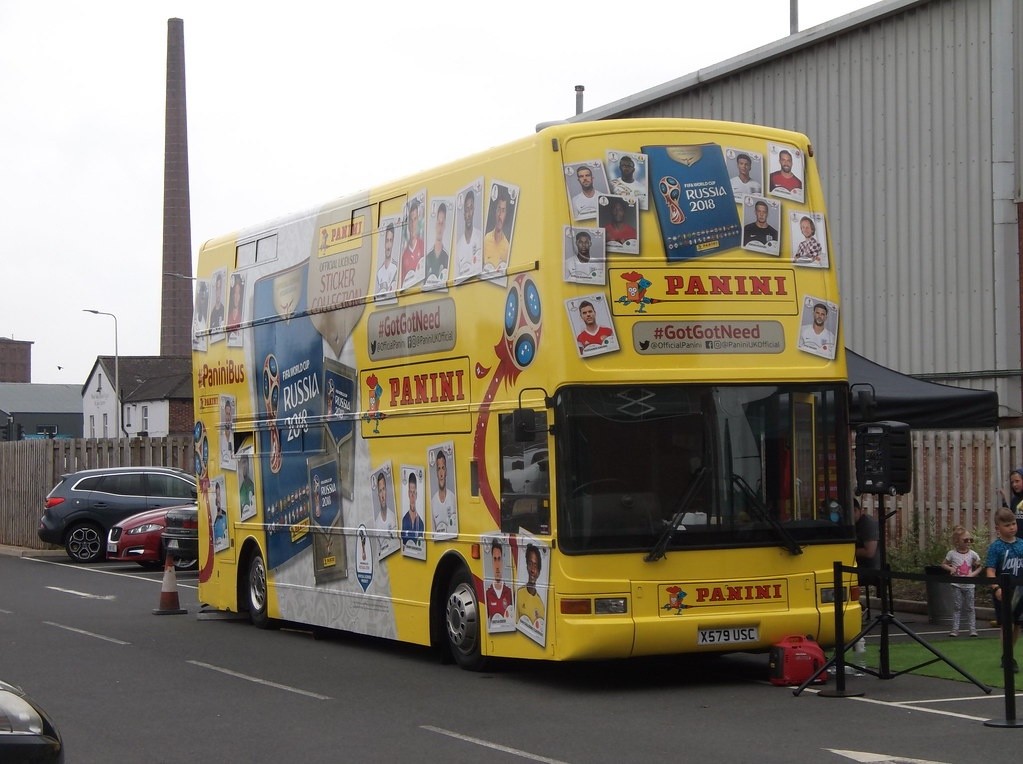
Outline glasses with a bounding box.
[961,538,974,543]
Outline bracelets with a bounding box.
[993,585,1000,592]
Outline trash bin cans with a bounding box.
[926,567,972,620]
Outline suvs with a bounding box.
[36,465,201,564]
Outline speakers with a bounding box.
[855,421,912,496]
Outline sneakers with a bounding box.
[949,627,959,636]
[969,628,978,636]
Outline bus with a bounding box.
[188,117,877,673]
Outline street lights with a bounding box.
[83,309,129,469]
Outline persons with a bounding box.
[566,156,646,279]
[373,473,398,554]
[798,304,835,356]
[939,525,983,637]
[193,268,243,348]
[983,467,1023,628]
[486,543,513,623]
[431,450,457,538]
[400,473,425,552]
[577,300,615,356]
[853,497,881,616]
[729,150,822,263]
[221,399,235,465]
[986,506,1023,674]
[378,190,512,300]
[210,482,229,547]
[515,544,545,630]
[238,455,256,519]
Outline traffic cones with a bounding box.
[151,550,189,615]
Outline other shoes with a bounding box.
[1001,655,1019,673]
[990,620,1001,627]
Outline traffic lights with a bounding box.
[1,422,12,441]
[13,422,25,442]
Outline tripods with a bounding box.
[793,494,992,697]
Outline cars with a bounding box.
[0,677,69,764]
[160,504,200,561]
[105,499,199,571]
